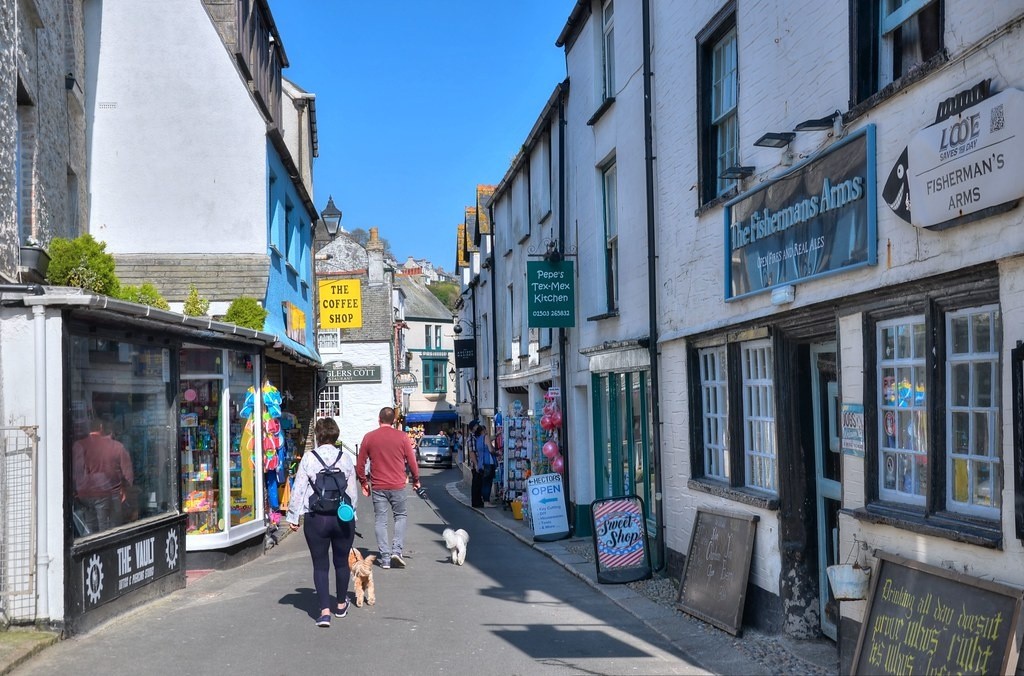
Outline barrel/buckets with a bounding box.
[511,502,522,519]
[826,540,872,600]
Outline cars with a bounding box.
[416,435,454,470]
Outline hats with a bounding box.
[469,420,481,428]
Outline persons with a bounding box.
[71,416,133,535]
[475,424,501,508]
[356,407,421,569]
[405,427,463,484]
[286,417,358,626]
[466,420,484,507]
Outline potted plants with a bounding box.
[18,241,52,279]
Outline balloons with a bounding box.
[543,441,558,458]
[553,455,563,472]
[540,403,562,430]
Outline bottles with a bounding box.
[230,438,241,488]
[198,429,210,450]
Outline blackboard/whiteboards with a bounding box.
[591,494,652,584]
[672,506,760,638]
[848,550,1024,676]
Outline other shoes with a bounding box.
[315,615,331,627]
[380,562,391,569]
[335,599,351,617]
[484,502,497,508]
[391,555,406,566]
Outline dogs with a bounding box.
[442,527,471,566]
[348,548,377,608]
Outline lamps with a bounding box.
[718,163,756,181]
[449,367,456,382]
[753,132,796,148]
[550,239,561,262]
[792,110,846,131]
[454,318,478,334]
[314,194,343,242]
[771,284,794,305]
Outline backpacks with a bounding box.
[308,450,352,516]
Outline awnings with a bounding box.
[404,412,457,423]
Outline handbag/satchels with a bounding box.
[482,463,496,478]
